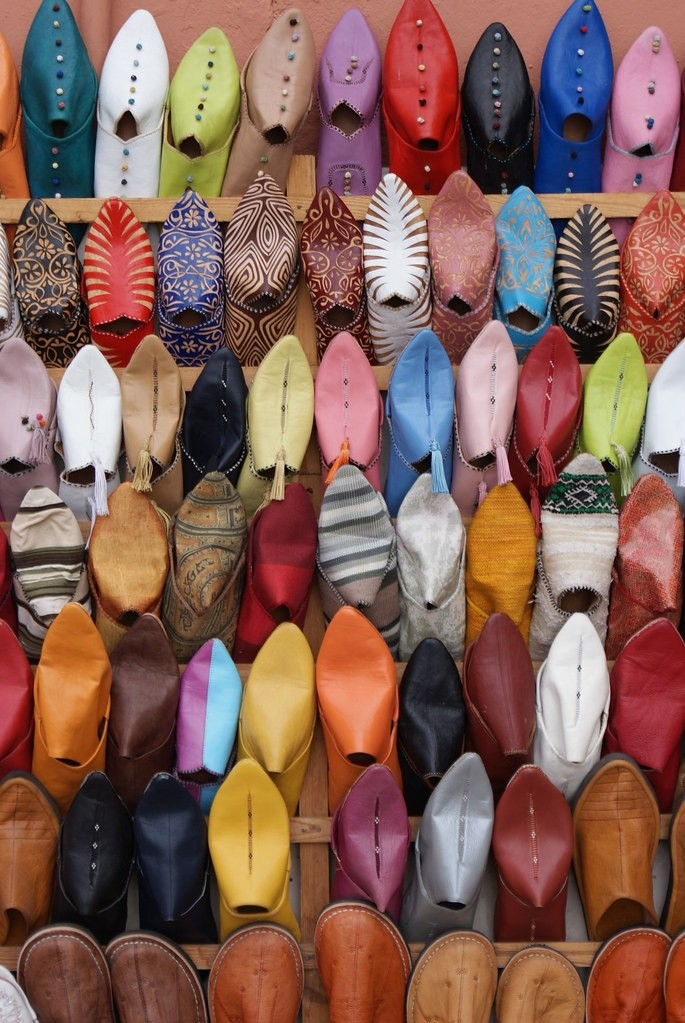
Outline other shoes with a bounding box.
[1,0,685,1023]
